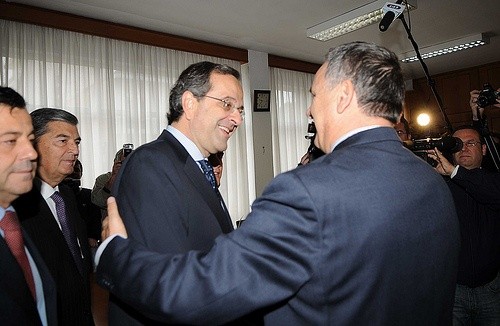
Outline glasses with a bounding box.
[465,140,482,148]
[194,94,246,120]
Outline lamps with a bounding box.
[399,33,488,64]
[305,0,418,42]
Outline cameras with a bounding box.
[119,144,134,164]
[477,83,500,108]
[305,122,324,160]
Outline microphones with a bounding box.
[378,0,408,32]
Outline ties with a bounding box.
[198,159,217,190]
[0,208,37,310]
[48,191,73,255]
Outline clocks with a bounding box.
[253,90,271,112]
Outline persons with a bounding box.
[396,118,412,142]
[59,159,101,258]
[0,86,59,326]
[93,41,458,326]
[470,90,500,176]
[427,124,500,326]
[108,60,266,326]
[90,148,133,220]
[11,108,95,326]
[298,154,309,168]
[208,151,224,187]
